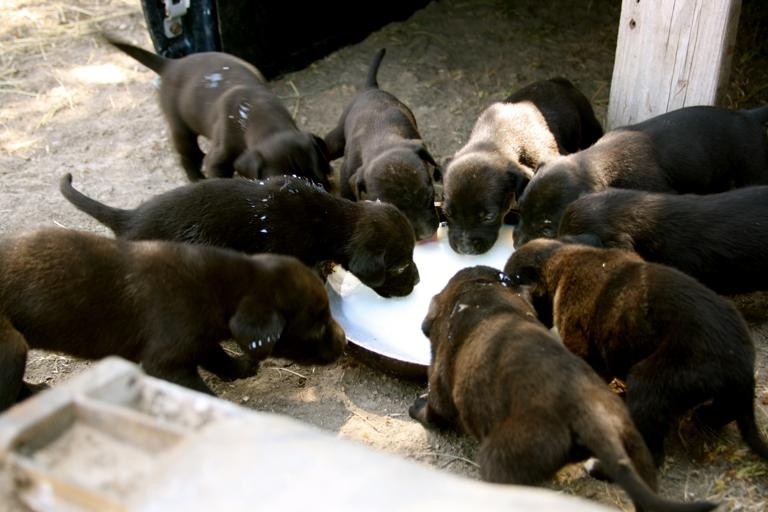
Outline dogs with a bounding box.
[59,173,419,301]
[432,74,606,257]
[88,25,336,189]
[557,179,768,298]
[0,227,346,414]
[500,103,767,248]
[501,237,768,485]
[325,47,442,242]
[408,264,720,511]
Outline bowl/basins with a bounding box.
[325,218,569,384]
